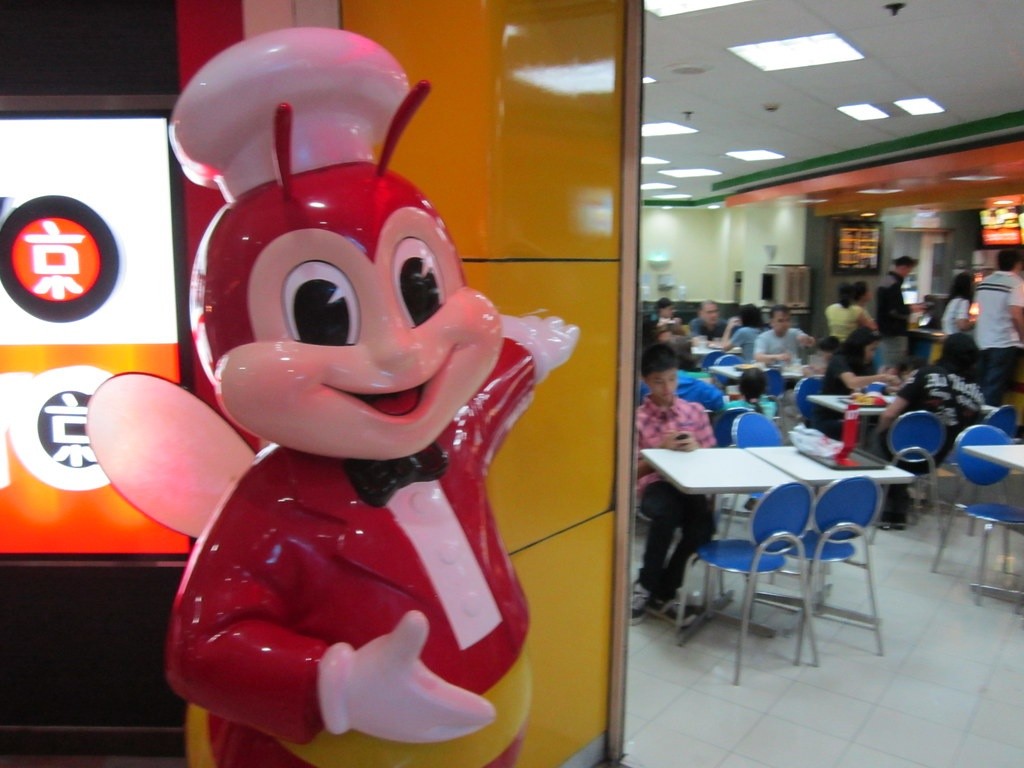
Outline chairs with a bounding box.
[641,301,1024,687]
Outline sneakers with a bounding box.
[648,592,697,628]
[630,580,648,626]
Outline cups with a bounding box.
[722,394,729,402]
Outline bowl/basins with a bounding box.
[789,430,844,458]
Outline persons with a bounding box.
[802,335,839,381]
[971,248,1024,408]
[857,332,989,528]
[691,300,739,350]
[752,305,816,388]
[878,252,927,374]
[627,343,727,625]
[721,302,769,366]
[643,298,695,372]
[826,283,878,347]
[812,327,904,441]
[940,270,979,365]
[717,368,779,509]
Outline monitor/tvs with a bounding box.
[832,220,884,275]
[975,207,1022,250]
